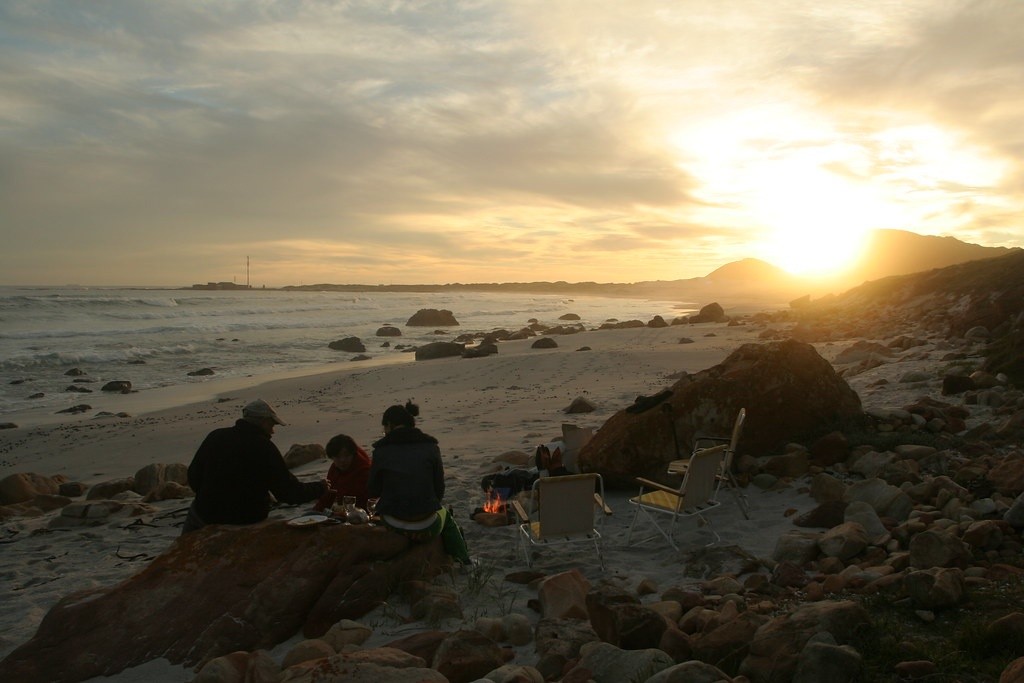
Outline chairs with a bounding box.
[667,408,749,520]
[625,443,730,555]
[511,473,614,573]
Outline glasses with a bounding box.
[382,429,385,436]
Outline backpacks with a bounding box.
[481,467,529,498]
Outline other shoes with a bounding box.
[461,558,481,574]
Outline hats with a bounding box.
[242,398,288,427]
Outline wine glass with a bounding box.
[367,499,379,525]
[342,496,356,525]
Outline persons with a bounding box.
[312,434,382,513]
[370,403,468,559]
[180,399,331,537]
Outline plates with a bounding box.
[287,514,328,527]
